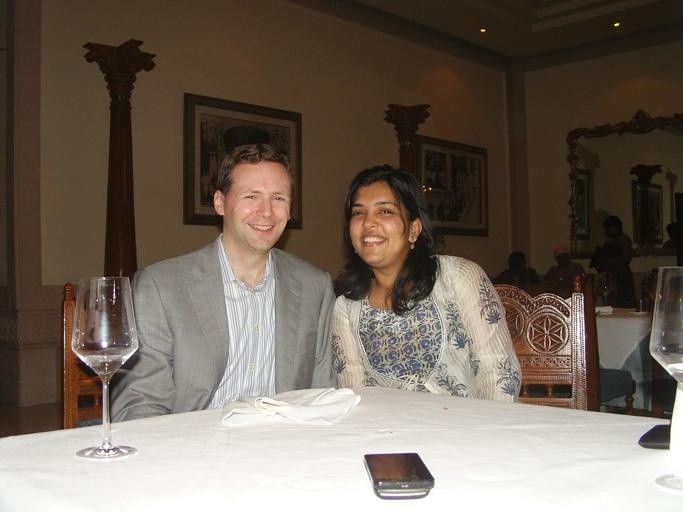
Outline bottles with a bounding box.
[637,296,645,312]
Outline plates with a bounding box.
[629,311,650,316]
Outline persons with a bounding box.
[596,215,639,308]
[660,221,678,250]
[106,141,337,425]
[543,245,585,283]
[329,163,525,402]
[494,251,538,288]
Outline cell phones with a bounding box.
[364,453,434,500]
[639,424,669,451]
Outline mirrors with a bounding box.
[564,108,683,269]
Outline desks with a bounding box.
[594,305,664,415]
[1,381,683,508]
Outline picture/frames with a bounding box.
[181,92,305,232]
[411,134,490,238]
[571,167,590,241]
[632,179,664,246]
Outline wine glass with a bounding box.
[69,276,141,459]
[598,271,615,307]
[649,266,683,492]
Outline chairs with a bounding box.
[59,284,105,429]
[494,285,587,412]
